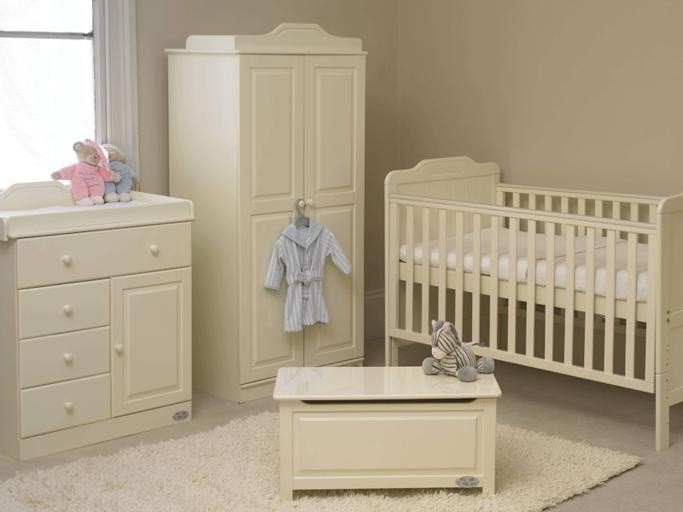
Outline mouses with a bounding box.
[292,199,310,232]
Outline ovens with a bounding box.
[382,153,682,453]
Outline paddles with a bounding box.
[0,408,645,512]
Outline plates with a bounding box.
[274,366,502,503]
[166,17,369,402]
[0,178,196,463]
